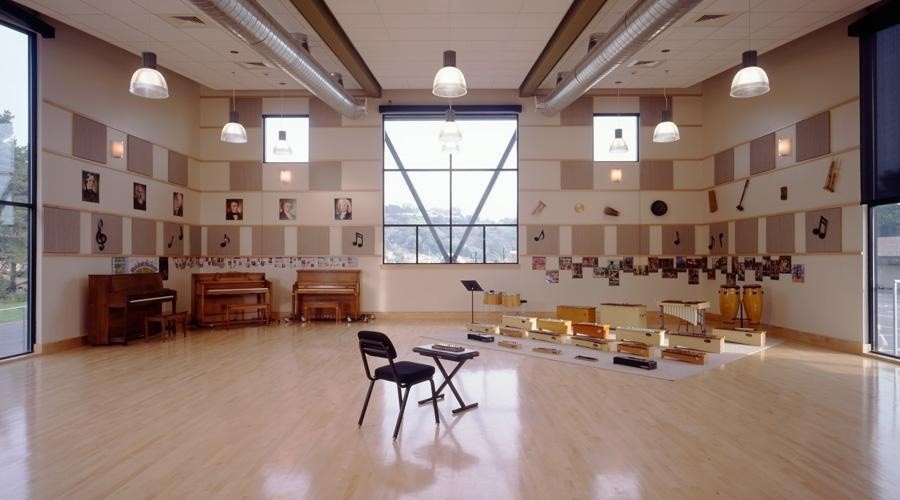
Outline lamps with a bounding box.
[730,0,770,100]
[129,13,170,100]
[777,137,791,156]
[611,168,623,183]
[273,83,293,156]
[111,134,123,159]
[280,167,291,185]
[653,70,680,143]
[609,82,628,155]
[432,51,467,98]
[438,98,464,153]
[220,71,248,144]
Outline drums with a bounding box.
[719,285,740,324]
[483,292,503,305]
[503,294,521,308]
[742,284,764,325]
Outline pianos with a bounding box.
[88,273,177,347]
[191,272,273,329]
[291,269,361,322]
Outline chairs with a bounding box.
[358,331,440,438]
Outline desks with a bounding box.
[413,343,480,413]
[656,299,710,334]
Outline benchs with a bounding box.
[221,303,269,328]
[305,300,342,325]
[144,311,187,343]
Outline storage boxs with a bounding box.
[556,305,596,323]
[600,303,647,330]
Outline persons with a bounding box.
[226,200,243,220]
[280,199,296,220]
[173,193,183,216]
[335,199,352,220]
[134,185,146,210]
[82,173,99,203]
